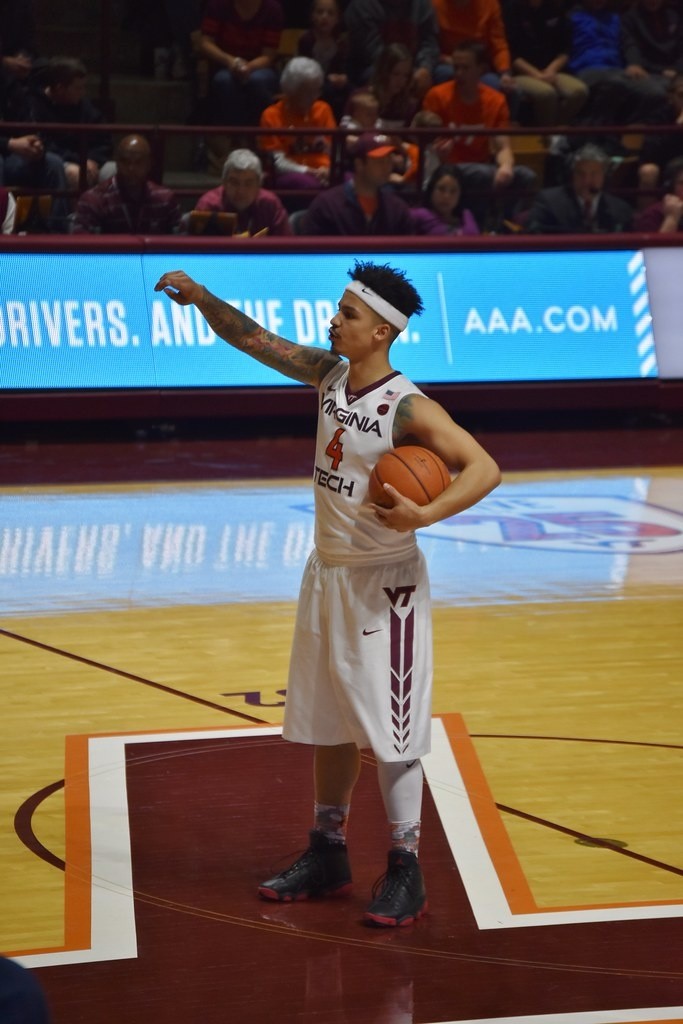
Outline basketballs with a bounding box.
[367,445,452,509]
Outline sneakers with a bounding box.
[255,829,353,904]
[363,848,428,927]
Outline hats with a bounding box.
[355,132,399,158]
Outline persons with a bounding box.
[0,0,683,237]
[155,259,501,927]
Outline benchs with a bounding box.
[0,0,683,234]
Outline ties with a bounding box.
[584,200,593,224]
